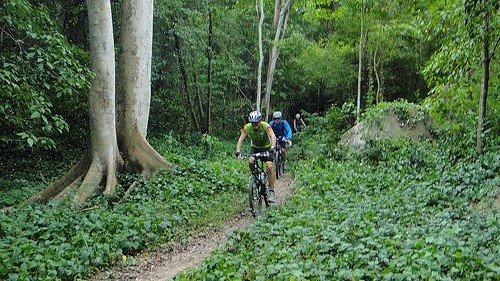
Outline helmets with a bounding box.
[247,111,264,123]
[296,114,301,119]
[273,111,283,118]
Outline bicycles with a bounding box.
[275,136,289,178]
[237,152,271,218]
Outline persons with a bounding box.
[235,110,277,202]
[269,111,293,170]
[293,114,306,132]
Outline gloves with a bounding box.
[268,146,276,156]
[235,152,242,160]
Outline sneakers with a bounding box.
[266,191,276,202]
[251,176,257,189]
